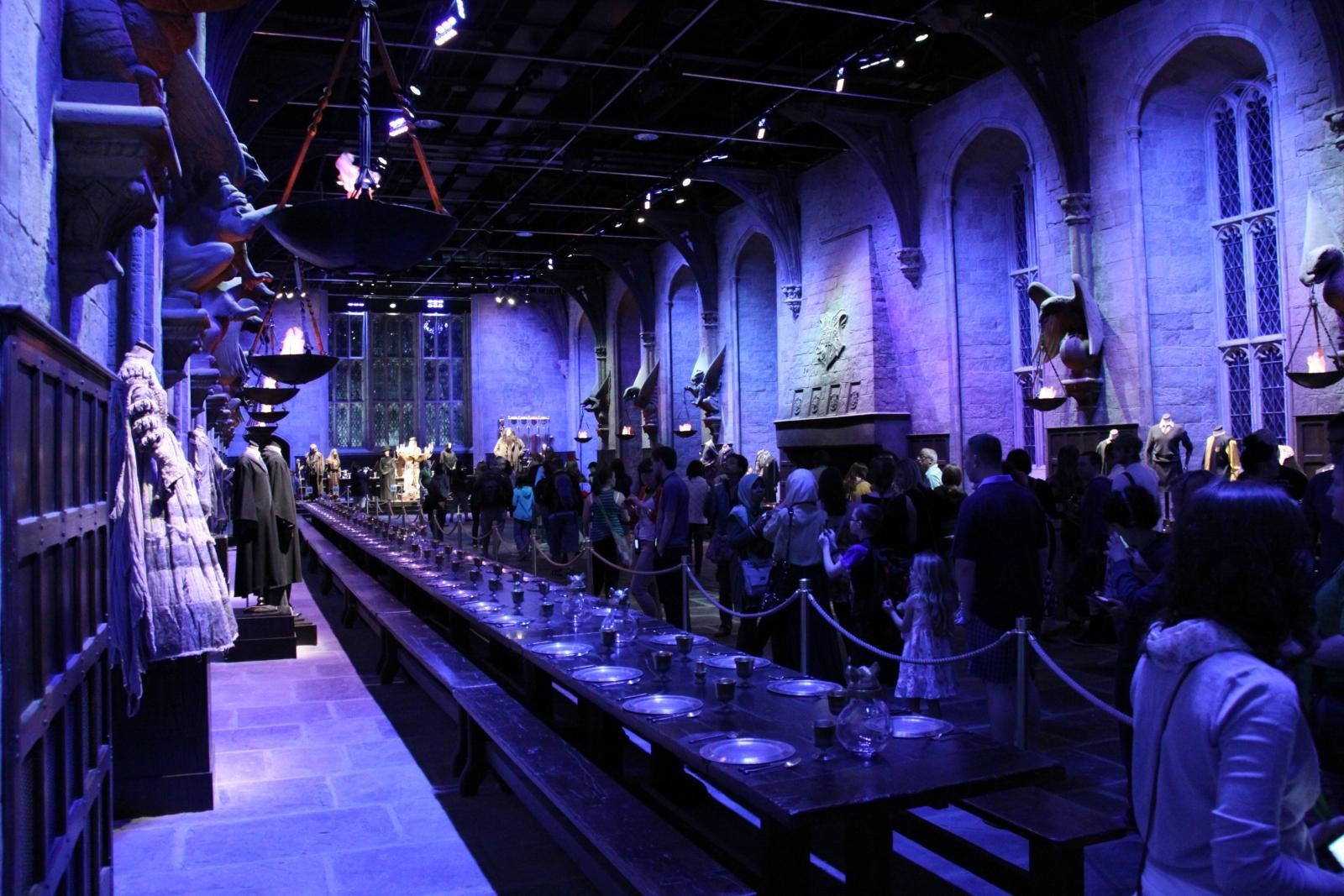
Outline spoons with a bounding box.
[321,501,973,777]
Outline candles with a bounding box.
[1306,351,1326,373]
[257,375,276,428]
[1040,385,1055,399]
[622,425,631,435]
[679,423,692,431]
[280,325,304,356]
[578,430,587,438]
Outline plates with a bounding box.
[306,500,954,773]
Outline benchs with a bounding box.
[293,492,1130,896]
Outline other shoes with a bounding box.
[1061,625,1116,646]
[714,625,730,637]
[517,551,528,561]
[551,570,558,578]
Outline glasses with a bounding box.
[918,454,931,459]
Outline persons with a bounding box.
[351,464,375,514]
[305,444,343,500]
[701,439,783,505]
[1096,414,1246,531]
[1129,484,1344,894]
[107,339,242,714]
[495,428,527,489]
[378,437,457,502]
[190,424,306,618]
[1237,409,1344,829]
[418,448,1225,756]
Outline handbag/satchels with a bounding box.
[613,529,639,568]
[740,557,773,599]
[760,557,790,610]
[705,532,740,562]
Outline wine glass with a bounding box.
[316,497,850,765]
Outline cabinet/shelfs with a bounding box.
[1,301,121,896]
